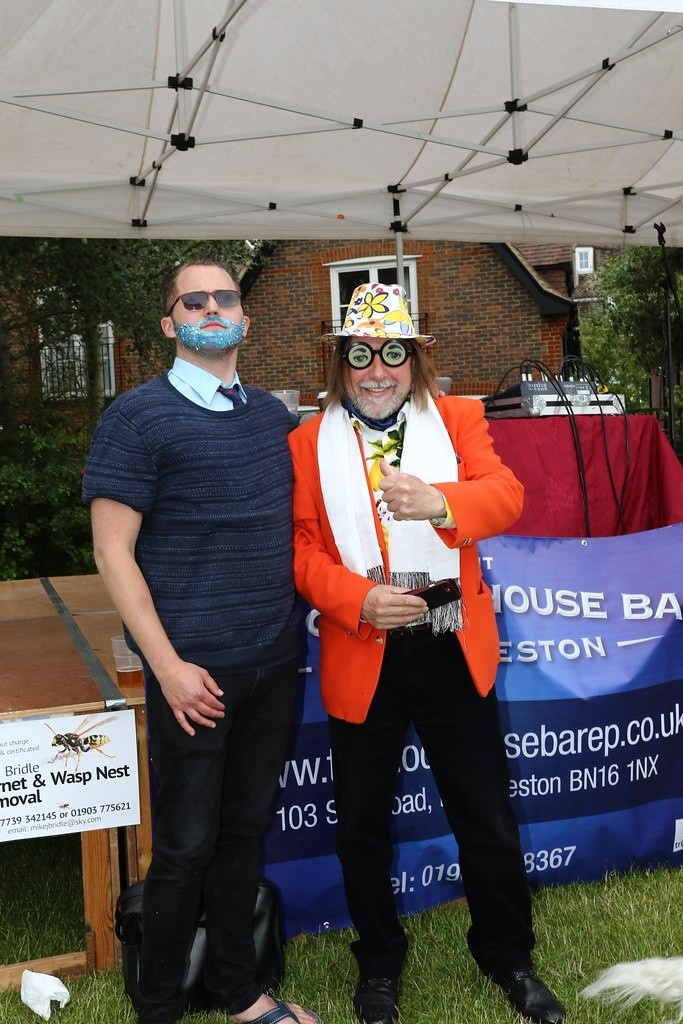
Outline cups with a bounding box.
[272,390,300,417]
[110,635,143,688]
[317,391,328,412]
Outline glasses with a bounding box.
[167,290,242,316]
[340,337,412,370]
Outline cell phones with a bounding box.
[402,578,461,611]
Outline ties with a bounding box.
[218,385,245,409]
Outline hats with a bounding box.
[320,283,436,349]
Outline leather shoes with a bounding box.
[496,966,565,1024]
[353,973,397,1024]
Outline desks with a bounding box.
[485,415,683,539]
[1,575,153,992]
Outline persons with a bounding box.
[288,282,570,1023]
[83,257,322,1024]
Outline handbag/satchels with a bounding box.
[114,877,284,1016]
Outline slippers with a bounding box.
[230,996,324,1024]
